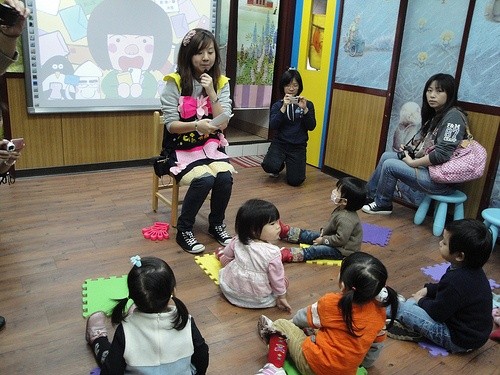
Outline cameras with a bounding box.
[1,138,25,157]
[0,3,20,26]
[289,97,298,104]
[397,145,415,160]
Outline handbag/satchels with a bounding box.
[425,109,487,184]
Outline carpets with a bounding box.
[229,154,266,168]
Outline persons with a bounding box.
[0,104,26,174]
[84,255,209,375]
[153,28,238,254]
[375,218,493,354]
[261,69,316,186]
[361,72,468,215]
[277,177,368,264]
[257,251,398,374]
[215,198,293,314]
[0,0,30,76]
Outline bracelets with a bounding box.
[0,29,20,38]
[195,119,198,131]
[210,97,219,104]
[3,160,17,168]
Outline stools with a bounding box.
[481,208,500,253]
[413,189,468,237]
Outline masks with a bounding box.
[330,190,336,205]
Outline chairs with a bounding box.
[153,111,187,227]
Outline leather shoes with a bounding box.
[128,304,136,315]
[85,310,109,344]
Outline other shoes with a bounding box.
[377,287,406,303]
[280,247,293,263]
[254,363,287,375]
[257,314,274,344]
[269,172,280,178]
[276,220,290,240]
[384,319,423,342]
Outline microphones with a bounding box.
[204,68,208,73]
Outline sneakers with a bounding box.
[171,225,206,254]
[207,222,234,247]
[361,202,392,215]
